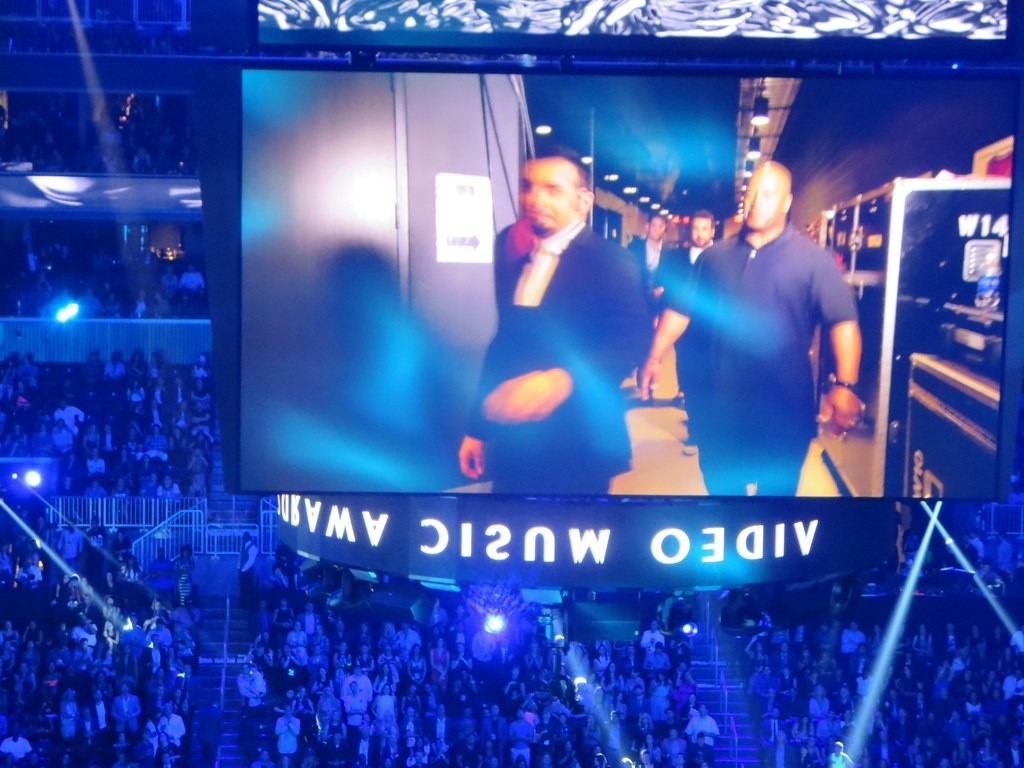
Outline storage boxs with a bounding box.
[940,303,1004,376]
[903,350,1000,502]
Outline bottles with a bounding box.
[975,252,1002,312]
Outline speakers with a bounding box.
[368,591,423,620]
[568,601,643,642]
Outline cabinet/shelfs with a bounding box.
[818,175,1009,500]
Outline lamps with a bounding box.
[736,95,770,214]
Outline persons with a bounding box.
[1,19,185,53]
[0,99,198,175]
[236,528,720,768]
[742,527,1024,768]
[0,349,214,513]
[0,515,202,768]
[641,161,861,495]
[0,242,210,319]
[459,144,655,496]
[653,209,715,405]
[628,211,669,399]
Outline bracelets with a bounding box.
[836,381,856,392]
